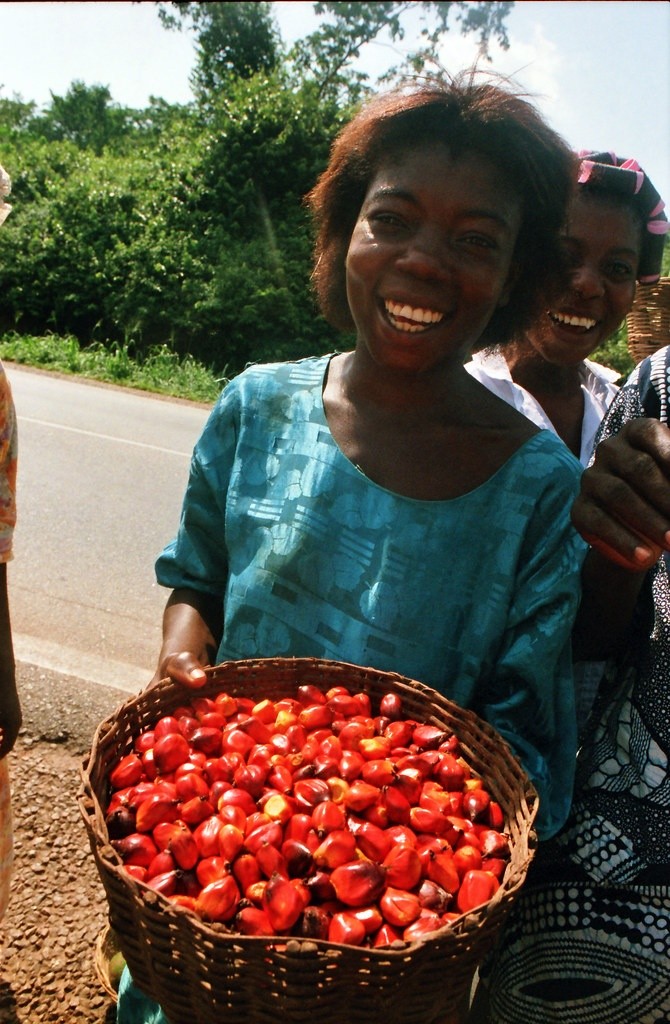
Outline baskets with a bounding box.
[93,925,127,1002]
[76,657,540,1024]
[626,277,670,364]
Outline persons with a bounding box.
[0,356,23,920]
[414,155,670,1024]
[116,64,583,1024]
